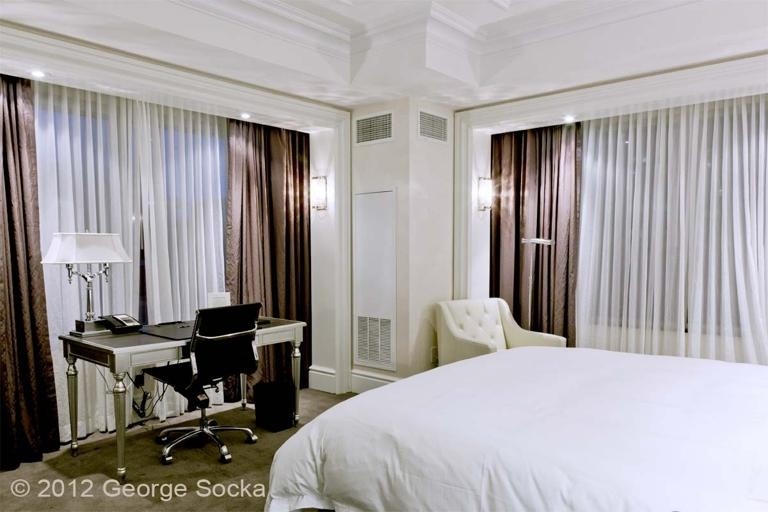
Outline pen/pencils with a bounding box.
[180,326,191,328]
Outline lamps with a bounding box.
[38,230,133,338]
[310,175,329,211]
[477,175,497,211]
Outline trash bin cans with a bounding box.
[252,381,297,433]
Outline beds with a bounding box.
[263,342,766,512]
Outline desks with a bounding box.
[57,313,311,485]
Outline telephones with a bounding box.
[98,314,143,334]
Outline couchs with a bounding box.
[432,294,570,367]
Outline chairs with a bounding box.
[140,299,261,466]
